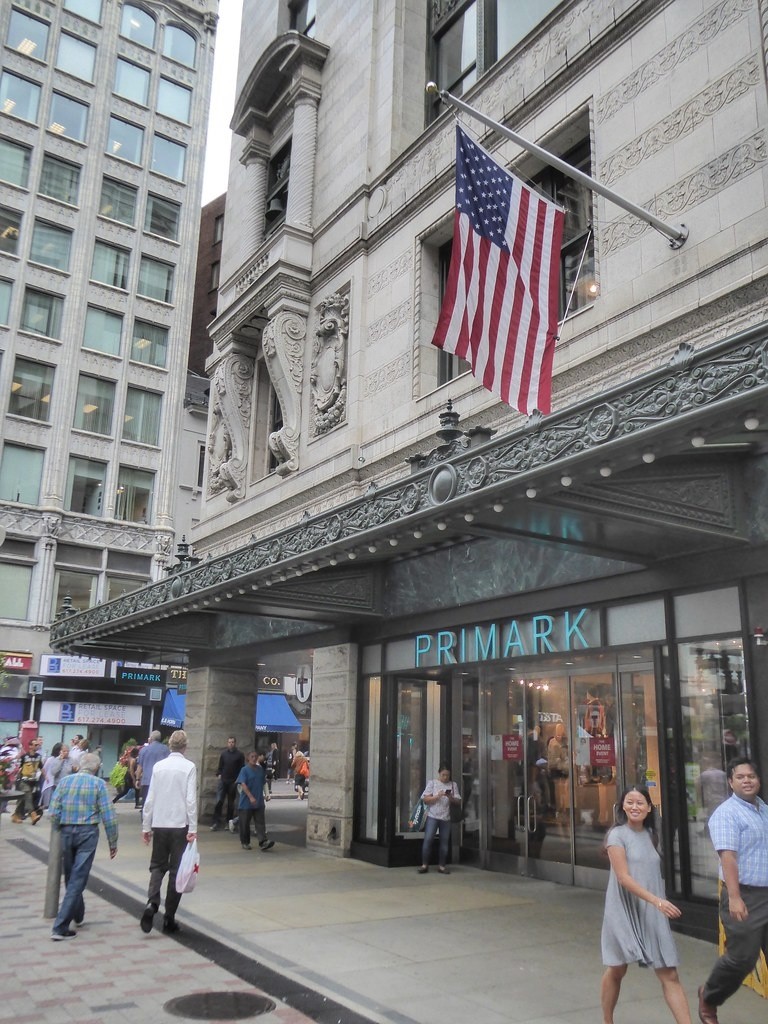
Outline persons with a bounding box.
[290,751,308,800]
[547,723,568,779]
[113,746,142,809]
[140,730,197,933]
[600,785,691,1024]
[48,753,118,939]
[696,757,727,837]
[209,737,244,831]
[286,743,299,784]
[10,735,102,825]
[698,755,768,1024]
[136,731,171,807]
[417,765,461,874]
[585,687,605,738]
[228,751,275,850]
[271,743,280,781]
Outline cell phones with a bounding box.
[445,790,451,793]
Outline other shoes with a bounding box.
[301,792,304,800]
[418,867,429,873]
[225,824,229,830]
[210,824,217,830]
[438,867,451,874]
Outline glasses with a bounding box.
[35,744,40,746]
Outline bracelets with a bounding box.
[659,898,662,906]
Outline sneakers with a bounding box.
[75,917,84,926]
[229,820,236,834]
[243,845,251,850]
[261,841,274,851]
[51,930,77,940]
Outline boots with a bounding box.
[12,814,23,823]
[31,811,41,825]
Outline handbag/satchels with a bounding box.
[176,838,199,893]
[409,799,430,831]
[451,805,463,822]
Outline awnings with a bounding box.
[160,689,303,733]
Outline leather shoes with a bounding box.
[163,918,179,934]
[698,985,718,1024]
[141,903,155,933]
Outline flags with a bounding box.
[431,124,566,417]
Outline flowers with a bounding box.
[0,756,22,791]
